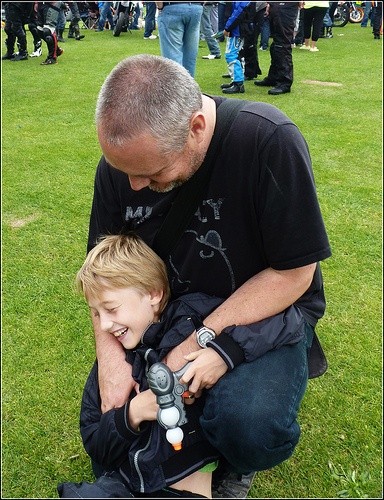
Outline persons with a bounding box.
[57,233,306,498]
[361,1,383,39]
[202,2,335,95]
[155,1,204,76]
[78,53,330,497]
[2,1,157,65]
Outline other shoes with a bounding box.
[222,74,231,77]
[268,86,284,94]
[202,54,221,59]
[222,85,243,93]
[95,29,102,32]
[255,79,272,85]
[310,47,320,52]
[299,45,310,50]
[221,84,231,88]
[144,34,157,39]
[374,35,380,39]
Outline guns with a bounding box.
[147,361,196,450]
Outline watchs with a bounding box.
[195,321,216,348]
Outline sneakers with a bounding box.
[211,462,257,499]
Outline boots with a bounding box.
[30,41,42,57]
[57,45,63,56]
[72,24,84,40]
[68,26,75,38]
[12,41,28,61]
[2,41,14,60]
[41,34,57,65]
[57,29,65,42]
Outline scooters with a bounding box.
[330,1,365,27]
[111,1,137,37]
